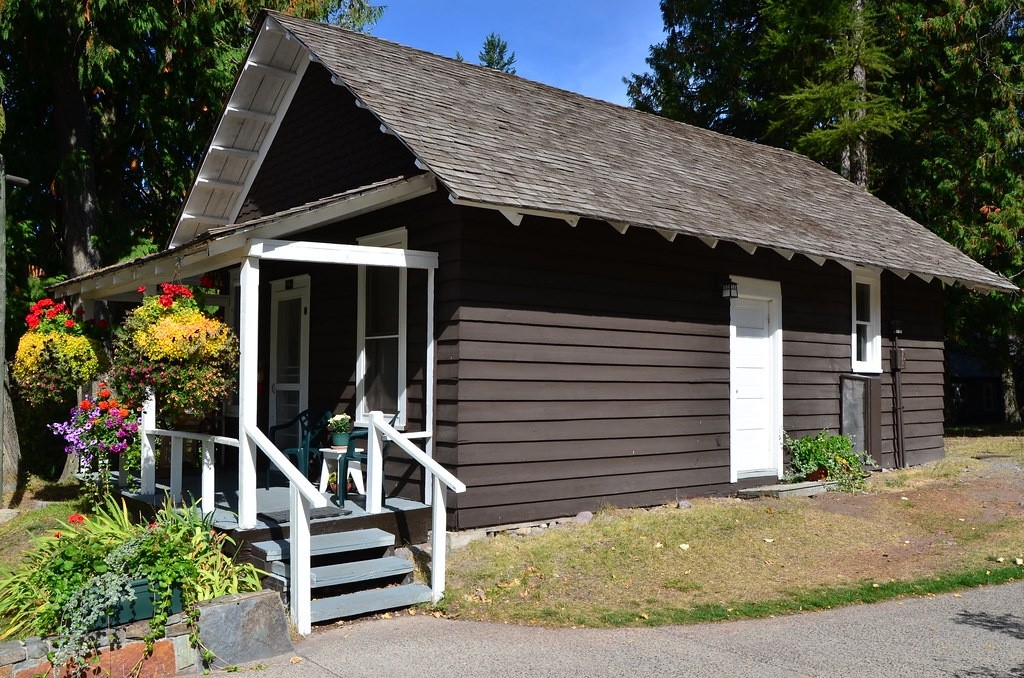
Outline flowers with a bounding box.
[12,301,99,408]
[328,412,351,433]
[112,284,238,420]
[47,373,141,497]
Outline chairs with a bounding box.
[338,410,399,509]
[265,409,332,491]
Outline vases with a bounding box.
[333,432,350,445]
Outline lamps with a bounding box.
[718,278,738,299]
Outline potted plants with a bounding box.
[778,425,877,493]
[328,467,351,495]
[0,491,262,677]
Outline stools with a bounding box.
[319,448,365,495]
